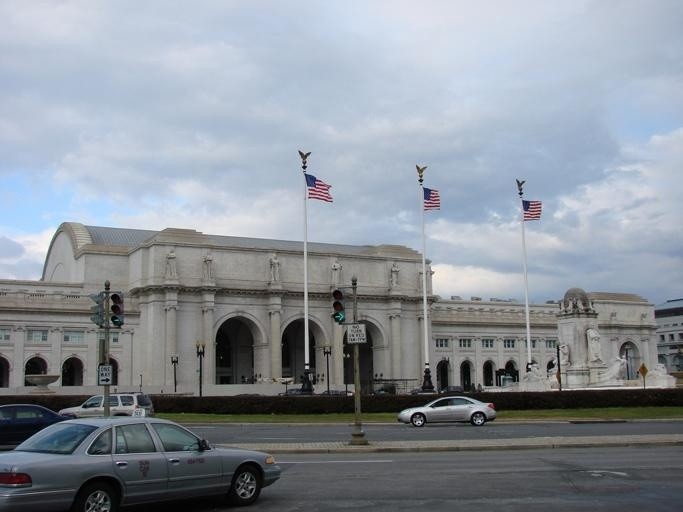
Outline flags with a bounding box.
[303,173,333,203]
[421,187,441,210]
[522,199,542,221]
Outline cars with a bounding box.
[278,388,354,397]
[397,396,497,427]
[417,386,464,395]
[367,388,386,396]
[1,392,281,512]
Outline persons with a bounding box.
[586,321,602,364]
[477,383,481,392]
[470,382,475,392]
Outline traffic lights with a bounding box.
[332,289,345,322]
[111,294,124,326]
[90,294,104,326]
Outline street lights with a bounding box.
[323,346,332,395]
[169,354,179,392]
[196,343,206,397]
[624,341,631,380]
